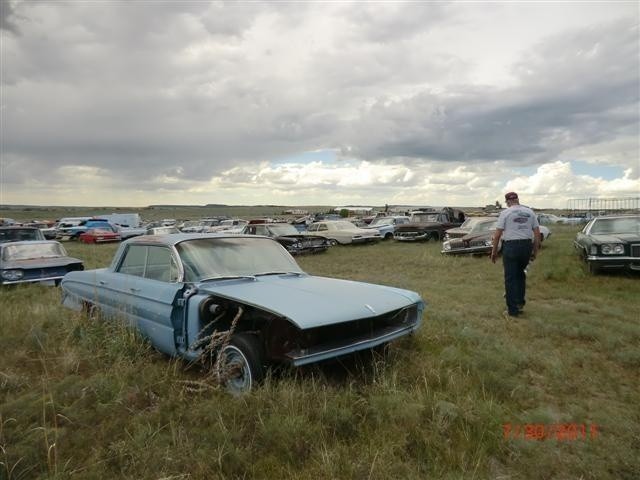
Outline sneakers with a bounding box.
[503,304,524,317]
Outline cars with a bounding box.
[0,240,84,290]
[0,207,596,256]
[575,214,640,273]
[57,230,427,405]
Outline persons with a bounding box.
[489,191,543,315]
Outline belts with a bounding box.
[502,239,532,244]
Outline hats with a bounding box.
[505,192,518,200]
